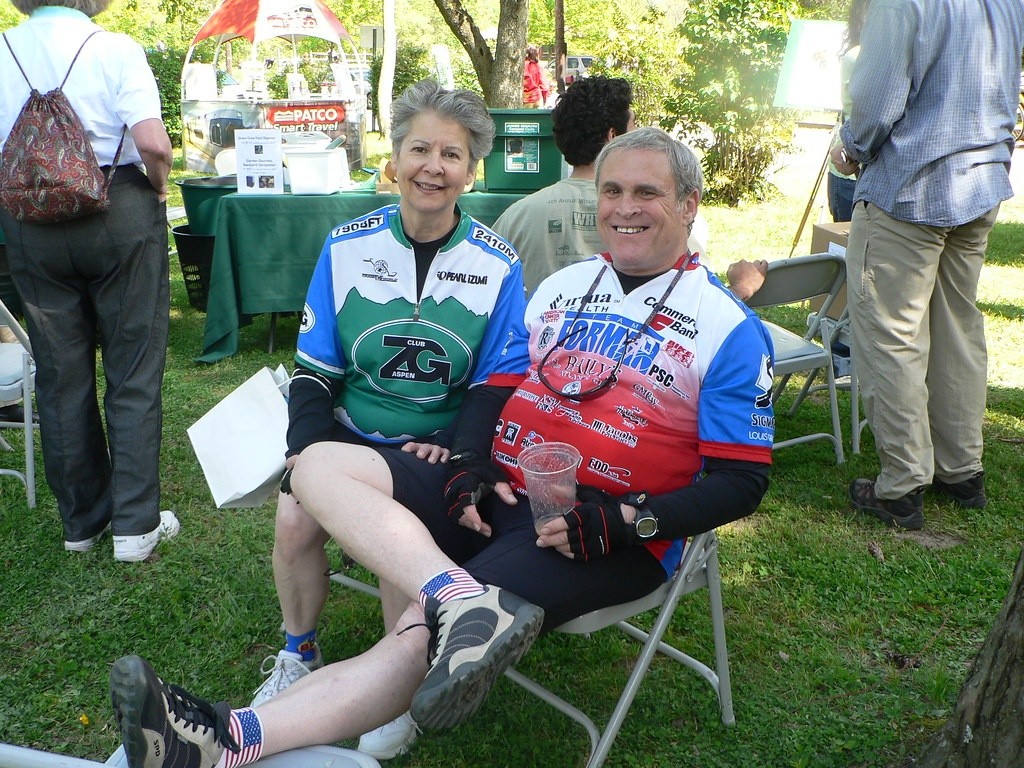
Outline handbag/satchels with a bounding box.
[187,363,336,509]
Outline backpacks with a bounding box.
[0,31,127,225]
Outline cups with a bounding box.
[518,442,580,536]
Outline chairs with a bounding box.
[746,252,847,464]
[787,301,870,454]
[0,302,37,508]
[499,529,734,768]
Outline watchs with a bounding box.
[633,504,661,538]
[840,146,850,165]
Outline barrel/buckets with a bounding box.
[175,176,237,235]
[171,225,215,313]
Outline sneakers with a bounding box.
[396,584,544,731]
[850,478,926,529]
[250,642,324,709]
[112,510,180,563]
[933,471,987,507]
[110,655,241,768]
[358,708,424,758]
[64,520,112,551]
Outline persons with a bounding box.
[107,125,774,768]
[830,1,1024,531]
[521,43,549,110]
[1,1,181,563]
[490,72,769,305]
[246,82,530,761]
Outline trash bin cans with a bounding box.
[173,175,238,294]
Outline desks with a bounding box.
[194,188,526,365]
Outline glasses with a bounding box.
[538,327,630,399]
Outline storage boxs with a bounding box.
[807,220,853,322]
[483,109,568,194]
[234,129,286,195]
